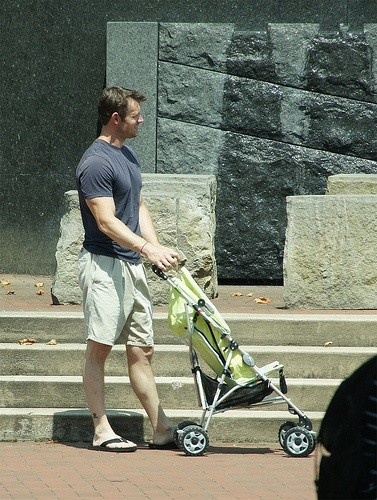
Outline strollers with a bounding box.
[151,254,320,459]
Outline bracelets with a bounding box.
[140,241,149,253]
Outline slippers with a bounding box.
[148,440,180,450]
[87,437,138,452]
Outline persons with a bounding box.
[76,85,187,452]
